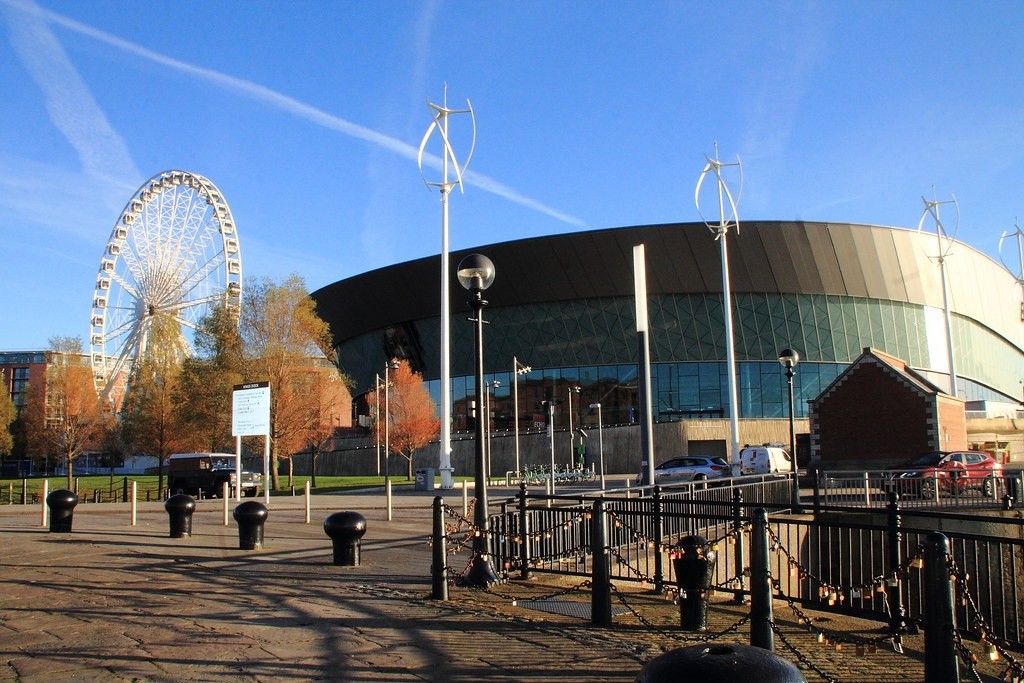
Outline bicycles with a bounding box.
[517,464,596,484]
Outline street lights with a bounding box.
[381,358,399,476]
[486,378,501,486]
[453,251,502,586]
[778,347,803,514]
[567,384,581,475]
[588,402,607,491]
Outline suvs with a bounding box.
[883,451,1003,498]
[637,455,730,488]
[738,446,798,478]
[167,452,261,498]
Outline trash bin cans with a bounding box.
[414,467,433,493]
